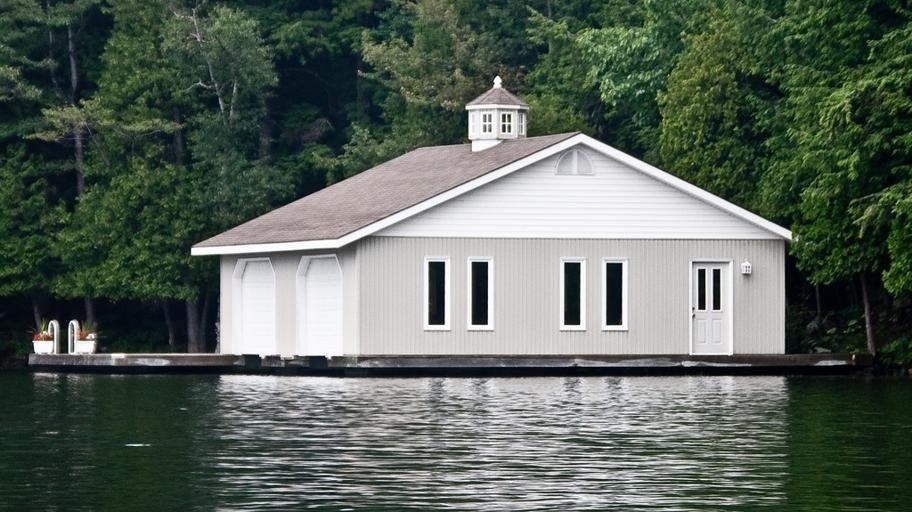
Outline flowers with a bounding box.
[72,321,101,339]
[27,319,54,341]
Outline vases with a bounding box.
[31,340,54,354]
[74,340,98,355]
